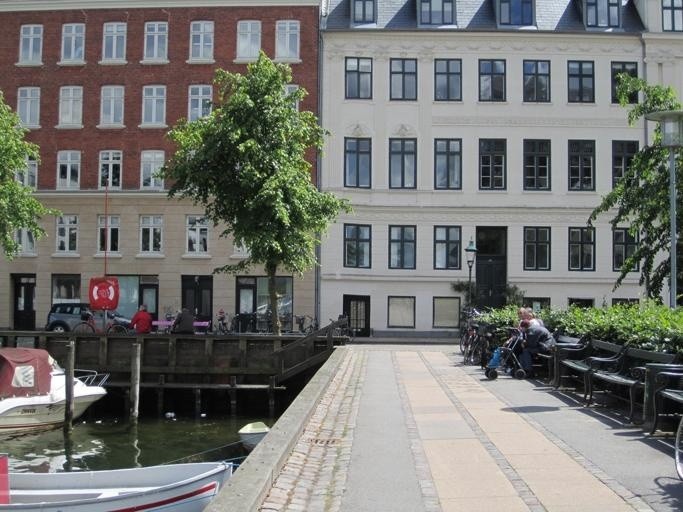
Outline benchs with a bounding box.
[538,326,683,484]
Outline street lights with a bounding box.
[646,109,682,309]
[464,239,479,312]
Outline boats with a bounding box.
[238,422,270,449]
[0,347,110,430]
[0,454,233,512]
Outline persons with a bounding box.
[485,307,555,378]
[128,304,151,336]
[169,305,193,333]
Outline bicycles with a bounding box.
[72,311,129,333]
[458,309,493,365]
[280,314,355,344]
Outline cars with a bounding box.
[45,303,136,333]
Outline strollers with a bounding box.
[485,328,529,380]
